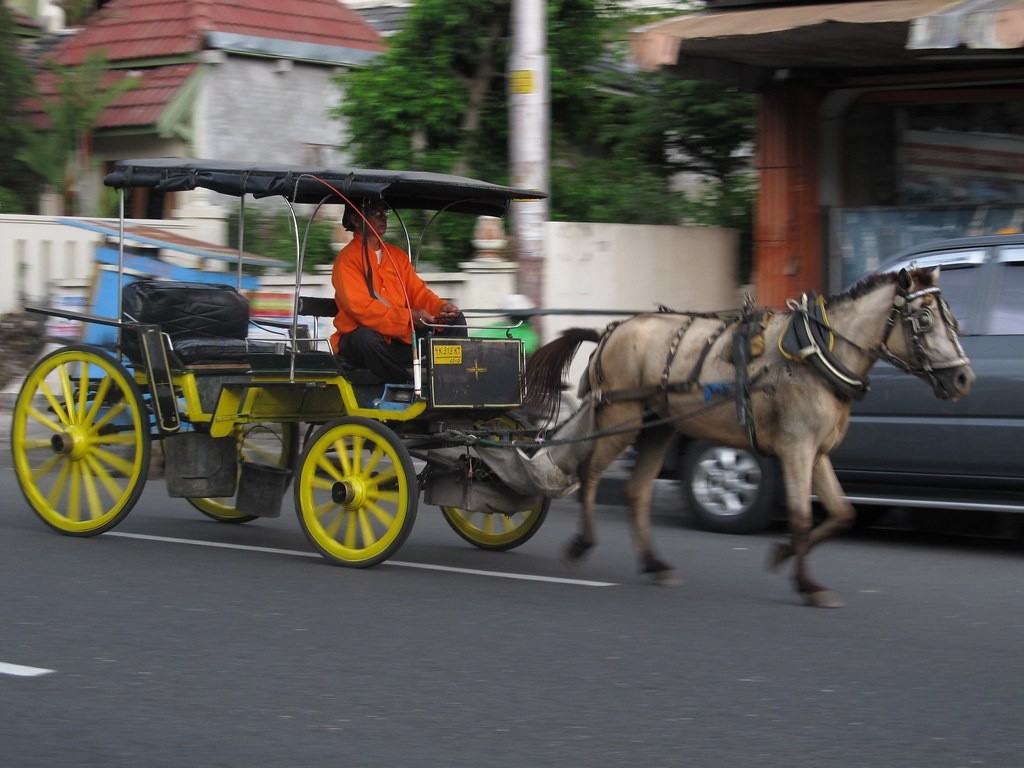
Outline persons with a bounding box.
[331,193,468,389]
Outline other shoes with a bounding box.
[392,390,413,402]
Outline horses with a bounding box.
[525,267,977,609]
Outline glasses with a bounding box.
[362,207,391,217]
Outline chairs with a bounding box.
[293,295,344,371]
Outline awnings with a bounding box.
[628,0,1023,118]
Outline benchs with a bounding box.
[121,282,259,377]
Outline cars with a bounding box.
[622,233,1024,534]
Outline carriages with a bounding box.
[10,156,975,609]
